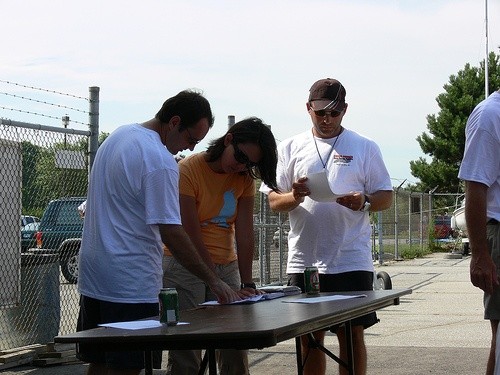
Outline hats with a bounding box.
[308,78,346,111]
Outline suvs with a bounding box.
[21,196,88,285]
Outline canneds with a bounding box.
[304,266,320,295]
[159,288,179,326]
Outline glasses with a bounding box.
[231,140,258,169]
[186,125,201,145]
[310,106,342,118]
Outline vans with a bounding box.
[418,214,453,239]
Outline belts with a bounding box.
[486,217,500,226]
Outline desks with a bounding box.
[54,289,412,375]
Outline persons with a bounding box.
[74,90,237,375]
[161,116,285,375]
[457,88,500,375]
[259,78,394,375]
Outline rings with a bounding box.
[350,204,353,207]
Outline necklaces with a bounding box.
[311,127,342,173]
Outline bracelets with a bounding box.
[240,282,256,289]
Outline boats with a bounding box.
[451,193,467,231]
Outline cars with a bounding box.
[370,223,379,237]
[272,220,291,248]
[20,215,41,231]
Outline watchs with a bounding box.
[359,194,371,212]
